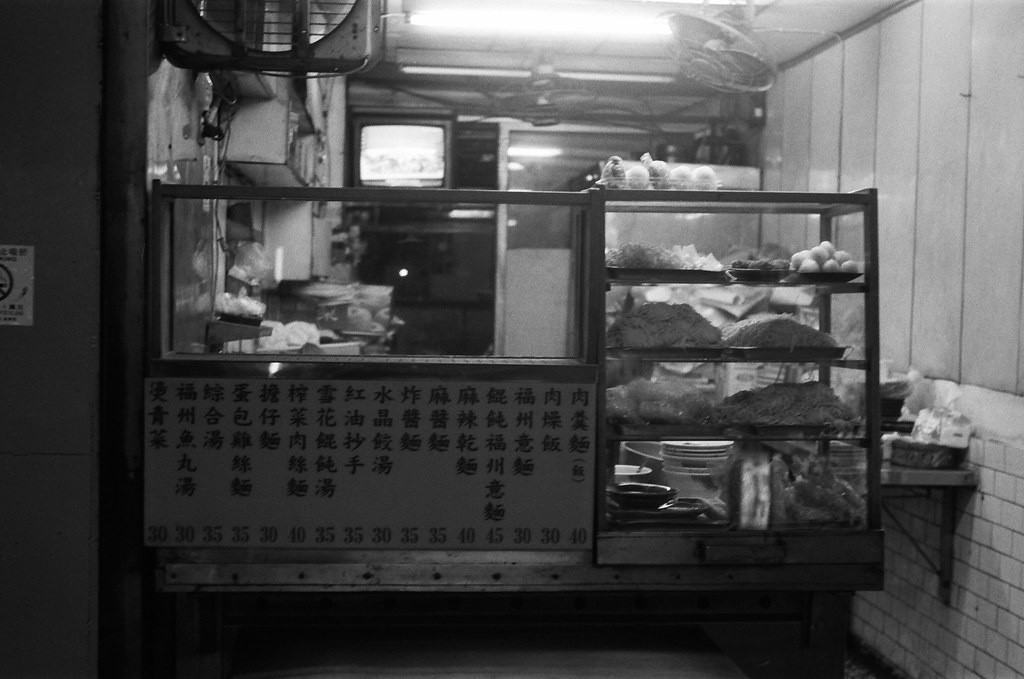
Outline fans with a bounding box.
[652,1,776,93]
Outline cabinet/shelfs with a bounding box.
[144,179,886,679]
[218,0,329,281]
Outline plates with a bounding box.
[608,498,708,520]
[658,442,735,471]
[725,266,797,280]
[793,271,864,285]
[859,397,905,422]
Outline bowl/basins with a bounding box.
[619,442,663,479]
[614,465,653,482]
[830,448,865,466]
[606,483,679,510]
[661,469,731,500]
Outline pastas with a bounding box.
[607,301,859,430]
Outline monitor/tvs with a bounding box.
[351,115,454,190]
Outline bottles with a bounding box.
[768,454,789,527]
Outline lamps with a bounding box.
[394,48,678,84]
